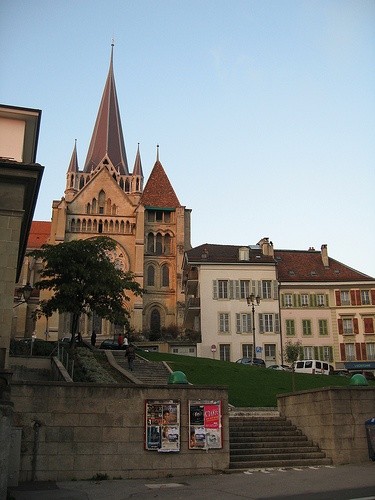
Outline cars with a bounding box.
[61,337,80,344]
[267,364,286,371]
[235,356,266,368]
[99,338,124,350]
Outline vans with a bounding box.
[280,359,350,378]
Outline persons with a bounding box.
[118,333,123,348]
[122,337,130,359]
[124,345,134,371]
[163,410,170,424]
[91,331,96,346]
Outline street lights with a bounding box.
[246,292,262,357]
